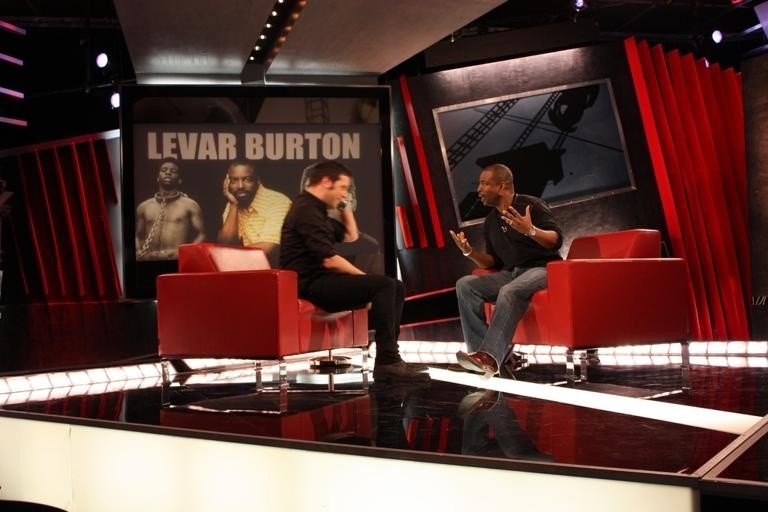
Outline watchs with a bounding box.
[525,225,538,238]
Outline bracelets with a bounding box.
[462,246,474,256]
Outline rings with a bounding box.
[509,221,513,225]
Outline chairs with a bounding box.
[157,241,372,411]
[469,228,692,388]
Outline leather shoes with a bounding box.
[457,351,495,378]
[369,383,430,400]
[373,361,429,382]
[459,389,498,418]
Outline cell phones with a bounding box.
[338,201,348,209]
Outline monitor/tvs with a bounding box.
[121,78,398,306]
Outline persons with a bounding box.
[133,155,206,261]
[349,380,432,449]
[214,156,294,267]
[275,158,431,382]
[451,384,558,461]
[300,160,382,272]
[449,161,566,377]
